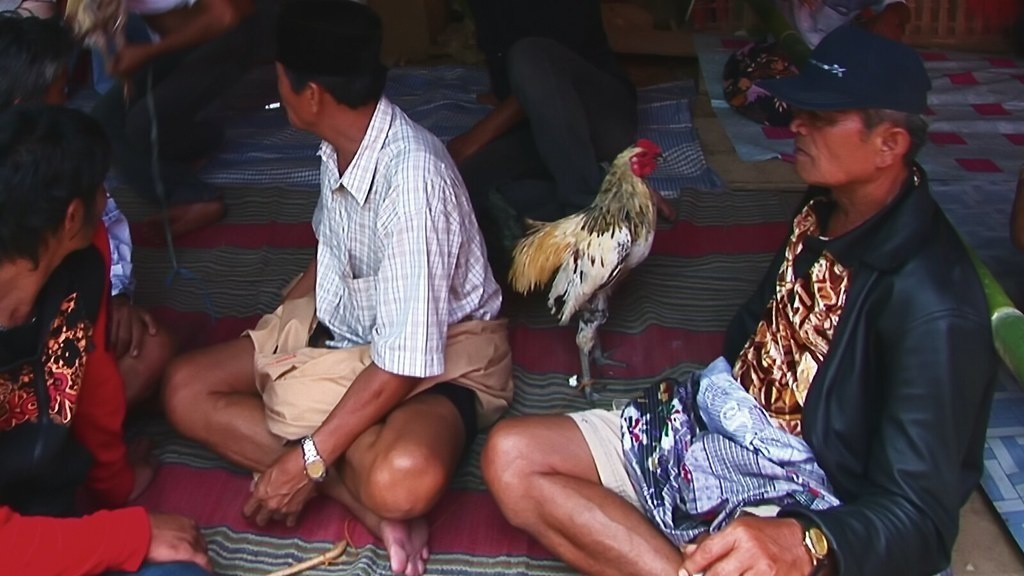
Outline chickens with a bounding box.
[508,138,663,410]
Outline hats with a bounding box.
[752,26,931,118]
[236,0,391,78]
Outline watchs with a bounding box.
[302,438,328,487]
[782,510,831,576]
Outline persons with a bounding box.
[722,0,911,126]
[1010,165,1024,255]
[0,0,255,576]
[165,0,512,576]
[481,27,994,576]
[446,0,676,285]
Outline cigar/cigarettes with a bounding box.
[265,102,281,109]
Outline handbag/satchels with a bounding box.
[618,356,842,548]
[722,41,808,125]
